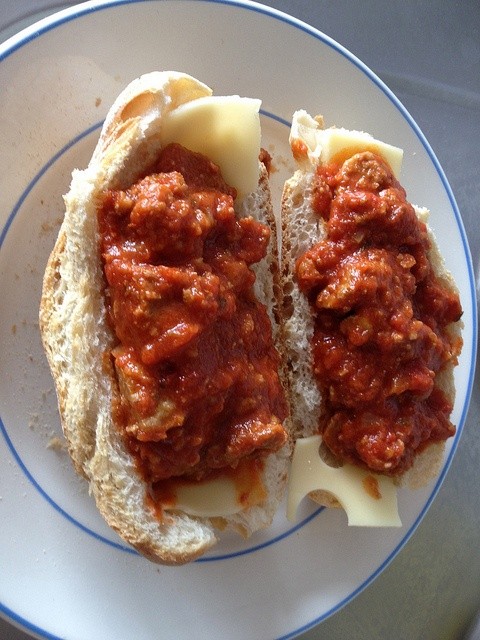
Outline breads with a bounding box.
[38,72,464,565]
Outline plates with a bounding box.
[1,1,479,640]
